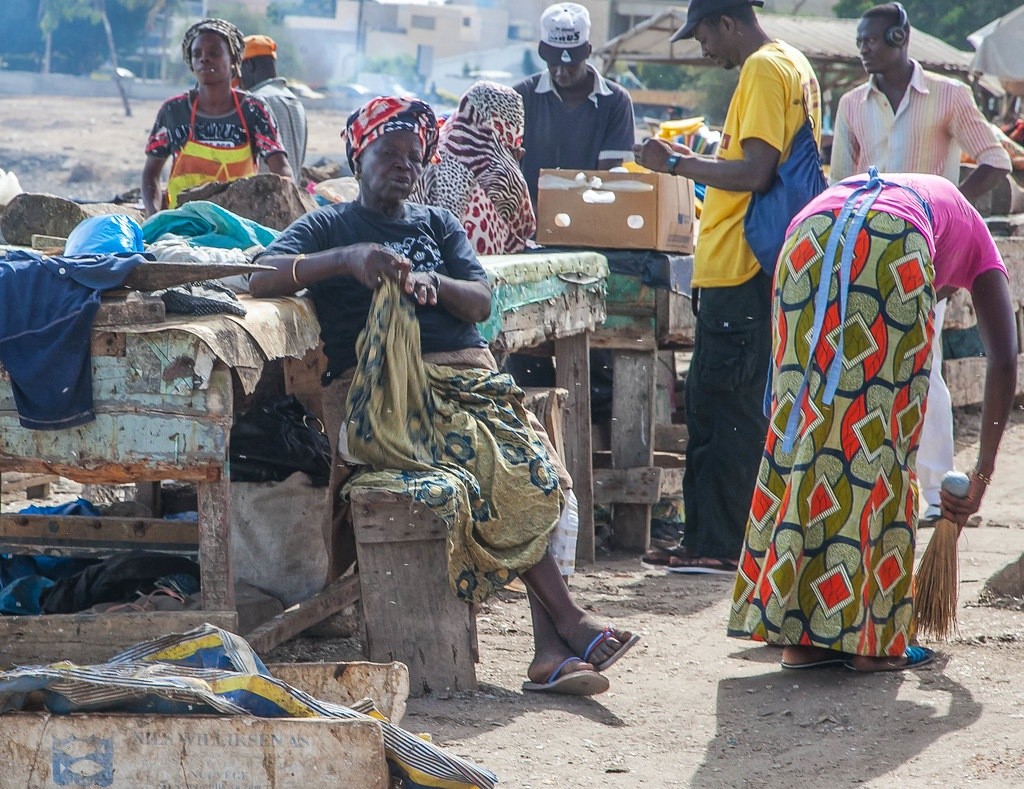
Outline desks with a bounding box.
[519,252,697,551]
[0,253,609,671]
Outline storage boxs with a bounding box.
[535,169,695,255]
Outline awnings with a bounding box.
[967,3,1024,97]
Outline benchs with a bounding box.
[349,386,571,697]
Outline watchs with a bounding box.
[667,154,681,176]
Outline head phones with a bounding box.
[884,1,908,47]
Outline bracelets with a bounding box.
[973,467,991,485]
[293,253,306,286]
[429,271,440,293]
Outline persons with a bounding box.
[728,165,1018,672]
[251,96,639,693]
[142,19,295,218]
[236,35,307,188]
[829,2,1013,200]
[407,2,636,525]
[632,0,821,573]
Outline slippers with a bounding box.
[583,625,641,671]
[641,544,738,574]
[781,644,936,673]
[522,657,610,695]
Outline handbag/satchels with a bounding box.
[744,120,830,278]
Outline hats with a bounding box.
[183,19,247,80]
[341,96,440,178]
[668,0,765,44]
[240,35,278,60]
[538,2,591,66]
